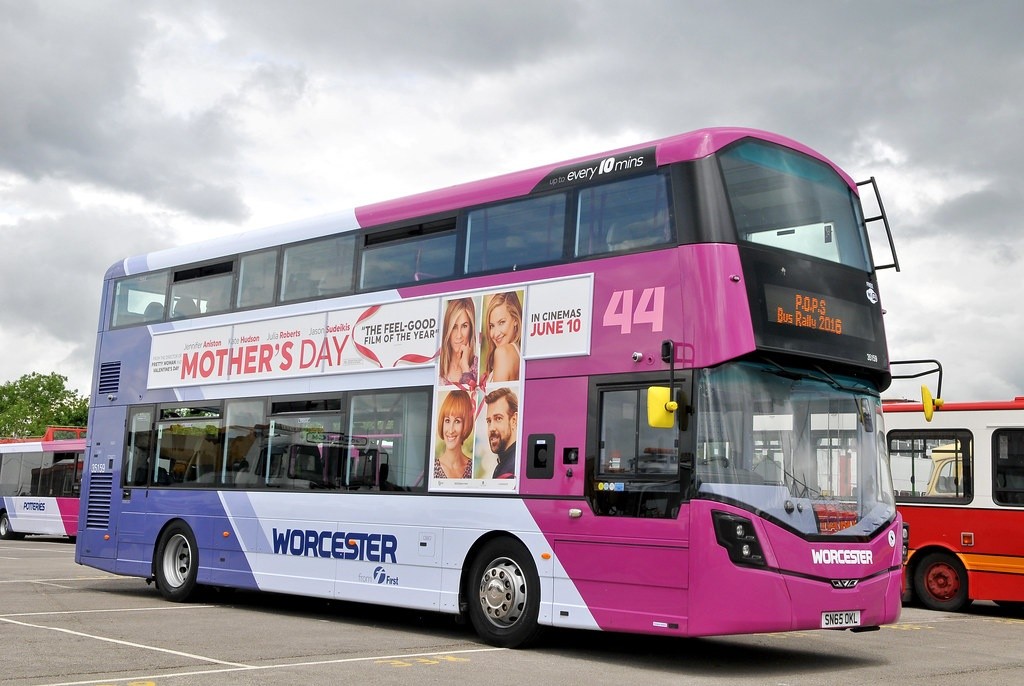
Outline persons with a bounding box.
[485,291,522,382]
[434,390,473,479]
[440,297,477,386]
[485,388,518,479]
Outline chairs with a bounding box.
[206,296,227,312]
[144,302,164,322]
[174,295,199,316]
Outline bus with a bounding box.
[76,128,943,649]
[227,433,405,493]
[0,425,87,541]
[750,399,1024,616]
